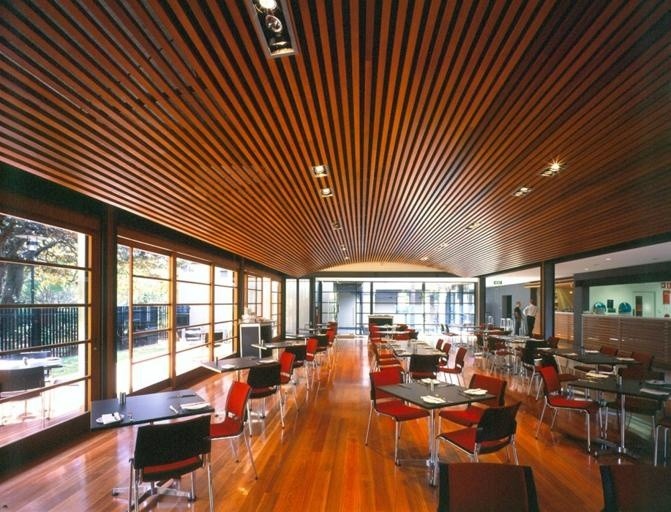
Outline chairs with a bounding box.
[0,366,46,418]
[19,351,50,377]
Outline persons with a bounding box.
[513,301,522,336]
[523,299,537,337]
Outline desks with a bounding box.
[0,357,63,420]
[90,389,214,502]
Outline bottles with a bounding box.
[120,392,126,406]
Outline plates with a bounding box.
[586,373,669,395]
[421,378,488,405]
[222,365,235,370]
[389,339,433,351]
[563,351,635,361]
[96,414,125,423]
[180,404,209,410]
[259,360,275,364]
